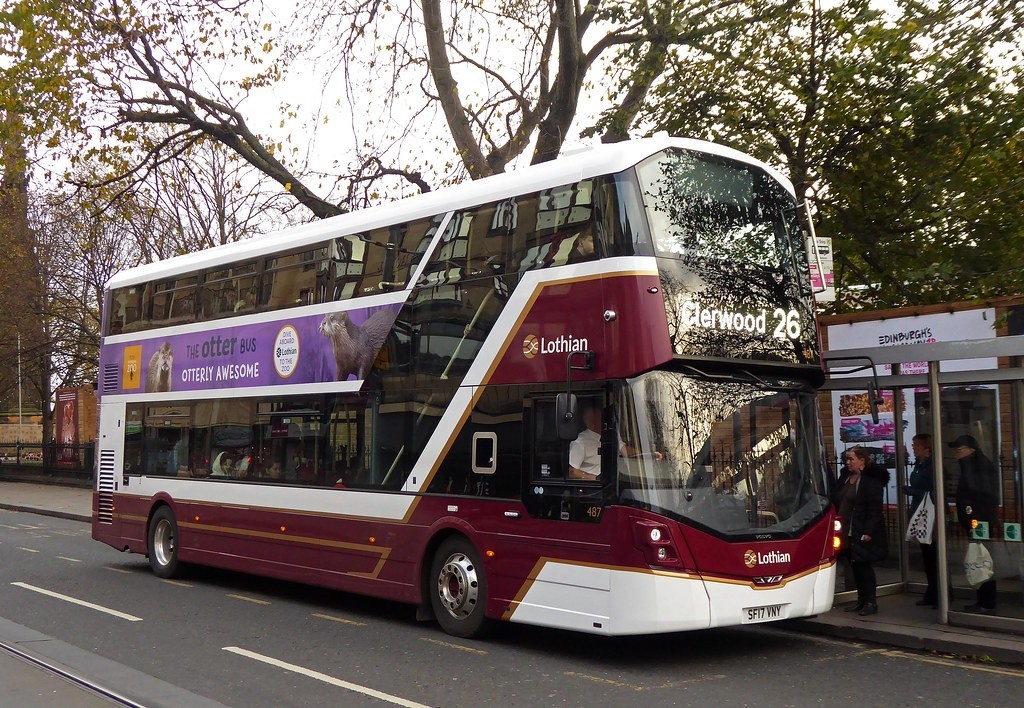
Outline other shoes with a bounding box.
[963,603,996,614]
[915,596,954,610]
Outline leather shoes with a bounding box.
[858,602,878,616]
[843,601,863,612]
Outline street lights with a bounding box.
[14,322,24,464]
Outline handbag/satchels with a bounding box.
[903,491,936,544]
[962,540,995,590]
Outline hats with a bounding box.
[947,435,979,450]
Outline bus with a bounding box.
[89,132,884,643]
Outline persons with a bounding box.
[569,408,662,481]
[902,435,955,610]
[570,230,596,264]
[840,447,890,616]
[946,436,999,614]
[173,423,368,485]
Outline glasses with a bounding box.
[954,444,970,453]
